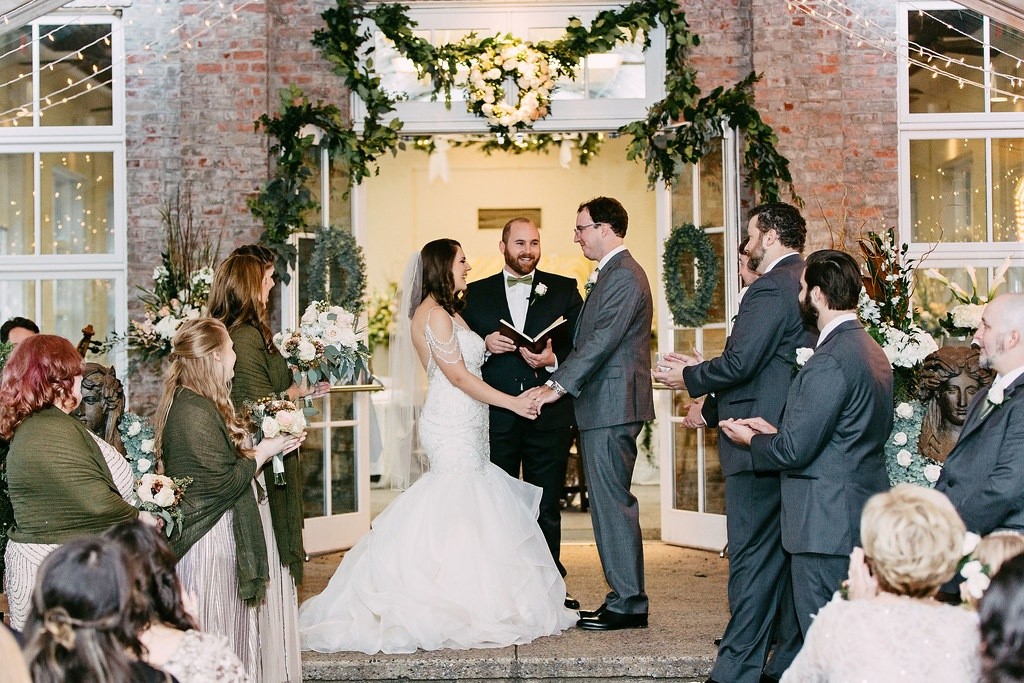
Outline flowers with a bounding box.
[119,410,194,536]
[832,185,937,407]
[956,557,992,599]
[790,347,814,378]
[925,255,1014,344]
[243,392,309,487]
[99,172,225,367]
[530,281,549,307]
[465,42,562,128]
[980,383,1012,419]
[584,270,599,294]
[267,301,375,385]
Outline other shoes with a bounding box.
[715,636,724,646]
[759,671,779,683]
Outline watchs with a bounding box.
[551,382,564,396]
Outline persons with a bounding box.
[0,622,32,683]
[297,238,581,657]
[529,196,656,632]
[933,294,1024,607]
[718,249,894,637]
[105,518,249,683]
[973,548,1024,683]
[20,537,179,683]
[652,202,820,683]
[205,244,331,683]
[0,335,139,634]
[463,218,587,609]
[963,530,1024,613]
[71,362,127,458]
[0,317,40,345]
[154,317,307,682]
[918,346,996,460]
[781,484,986,683]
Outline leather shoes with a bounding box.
[579,601,610,617]
[577,607,650,630]
[564,596,581,610]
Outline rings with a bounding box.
[529,361,532,364]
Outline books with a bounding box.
[499,315,567,354]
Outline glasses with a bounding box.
[574,222,610,236]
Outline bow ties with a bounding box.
[506,274,532,287]
